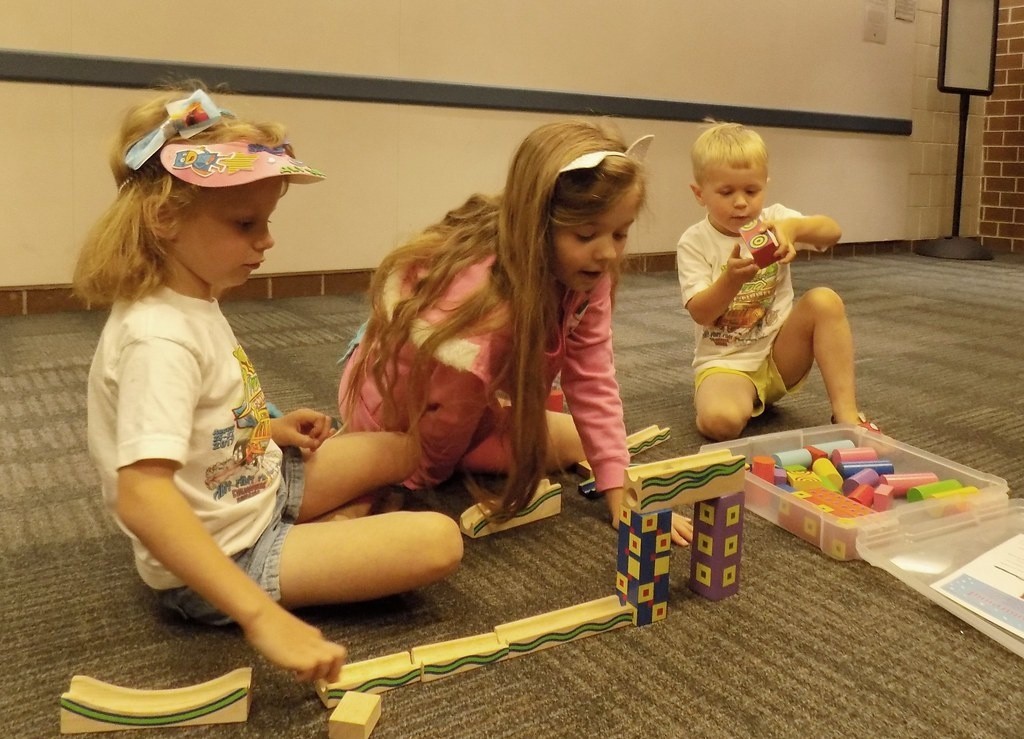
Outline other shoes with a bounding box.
[830,411,882,434]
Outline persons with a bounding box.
[675,122,898,452]
[338,121,694,547]
[70,89,463,686]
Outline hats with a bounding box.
[118,140,327,188]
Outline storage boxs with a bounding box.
[698,422,1024,660]
